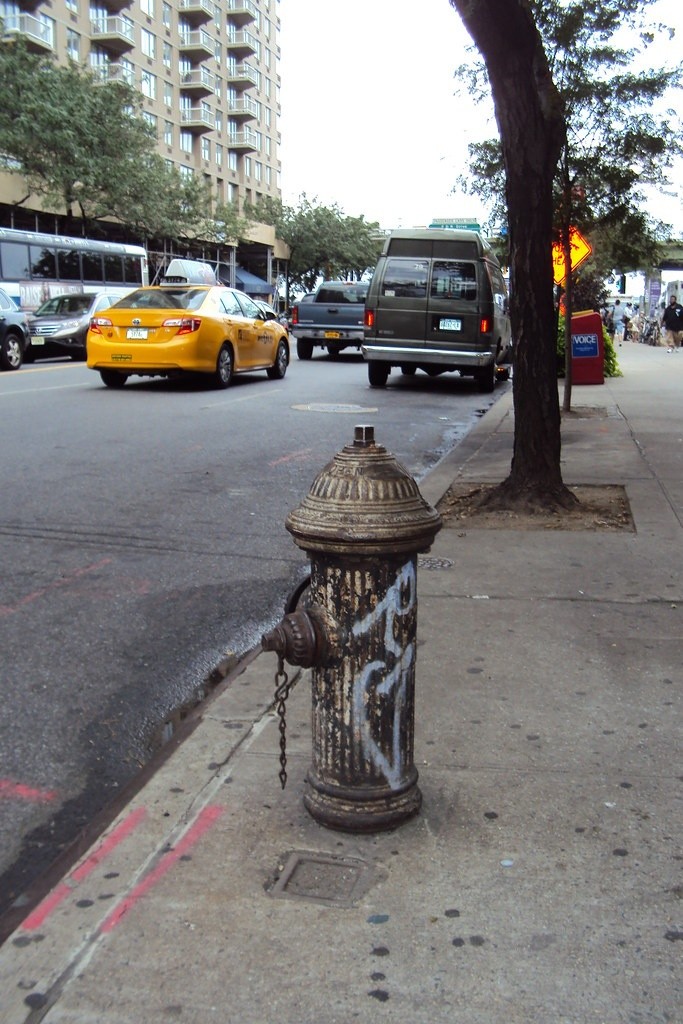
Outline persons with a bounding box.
[602,302,611,323]
[628,312,645,343]
[623,302,639,341]
[660,295,683,352]
[606,300,624,347]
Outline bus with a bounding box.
[0,226,151,319]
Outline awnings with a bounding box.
[218,265,273,293]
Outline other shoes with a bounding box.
[668,348,673,353]
[619,341,622,347]
[675,348,679,352]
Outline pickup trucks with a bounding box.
[290,279,371,361]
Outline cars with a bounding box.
[24,291,139,363]
[252,299,289,336]
[0,286,31,370]
[85,258,291,390]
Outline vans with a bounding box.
[359,226,510,394]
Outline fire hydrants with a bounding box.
[257,423,445,833]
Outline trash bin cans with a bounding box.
[570,309,604,384]
